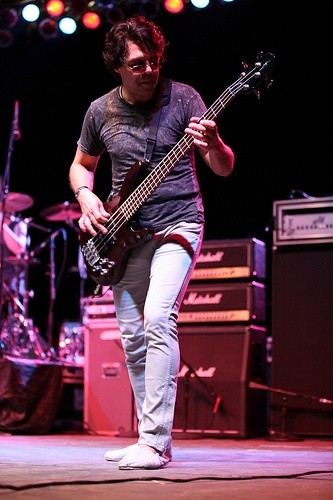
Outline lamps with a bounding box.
[0,0,102,47]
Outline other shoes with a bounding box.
[104,442,173,470]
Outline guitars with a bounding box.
[80,46,277,286]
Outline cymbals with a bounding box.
[41,202,82,221]
[0,192,33,212]
[4,256,41,265]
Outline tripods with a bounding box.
[0,237,71,365]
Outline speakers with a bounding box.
[168,241,332,438]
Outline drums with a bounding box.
[60,321,84,383]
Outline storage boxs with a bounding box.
[81,237,265,441]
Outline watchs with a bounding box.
[74,186,89,198]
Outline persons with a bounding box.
[0,181,32,318]
[68,17,234,470]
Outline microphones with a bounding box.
[30,228,60,255]
[12,101,20,140]
[212,395,223,414]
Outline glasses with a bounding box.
[119,55,160,72]
